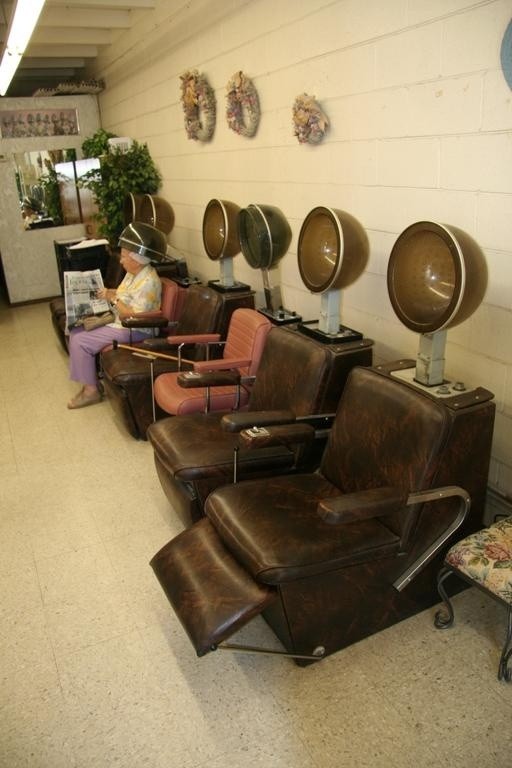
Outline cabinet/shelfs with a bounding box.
[73,158,110,240]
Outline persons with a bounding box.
[22,206,39,229]
[67,247,162,409]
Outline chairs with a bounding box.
[67,221,194,396]
[50,192,169,358]
[100,200,232,441]
[148,203,297,428]
[148,205,374,530]
[145,218,493,668]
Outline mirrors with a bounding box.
[11,147,84,231]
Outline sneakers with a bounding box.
[68,383,104,409]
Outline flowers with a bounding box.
[178,69,330,147]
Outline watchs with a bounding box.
[112,298,119,307]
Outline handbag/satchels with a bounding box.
[81,310,115,331]
